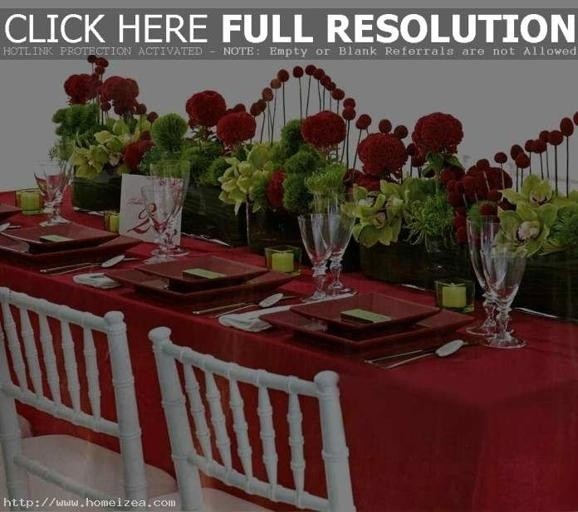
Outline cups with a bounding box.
[263,244,302,278]
[103,208,120,234]
[15,188,44,215]
[433,276,476,313]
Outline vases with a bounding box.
[300,234,578,320]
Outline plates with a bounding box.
[0,202,23,221]
[257,308,478,351]
[288,291,442,333]
[103,268,295,306]
[133,254,270,288]
[0,234,143,266]
[0,223,120,248]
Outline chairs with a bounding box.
[0,286,358,512]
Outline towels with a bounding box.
[72,271,122,290]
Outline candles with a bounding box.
[20,192,40,210]
[272,252,294,274]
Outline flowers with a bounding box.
[43,55,578,256]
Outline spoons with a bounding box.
[381,339,465,370]
[50,254,126,277]
[211,292,284,318]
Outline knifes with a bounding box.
[38,256,140,275]
[193,293,294,320]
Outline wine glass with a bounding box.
[465,214,527,350]
[297,194,359,303]
[30,136,75,230]
[140,158,190,266]
